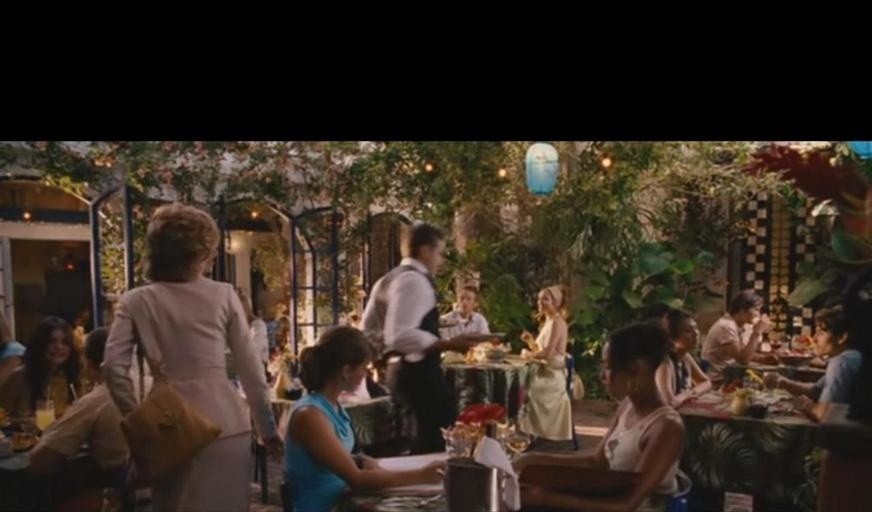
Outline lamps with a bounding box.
[524,140,559,193]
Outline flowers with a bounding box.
[457,402,506,455]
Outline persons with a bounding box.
[101,200,284,512]
[0,286,872,509]
[358,224,474,456]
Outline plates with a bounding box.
[0,456,32,471]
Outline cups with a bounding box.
[36,399,56,434]
[443,415,531,460]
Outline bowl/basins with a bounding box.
[485,349,507,359]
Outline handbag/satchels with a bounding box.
[120,385,222,481]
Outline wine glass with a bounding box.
[763,371,779,398]
